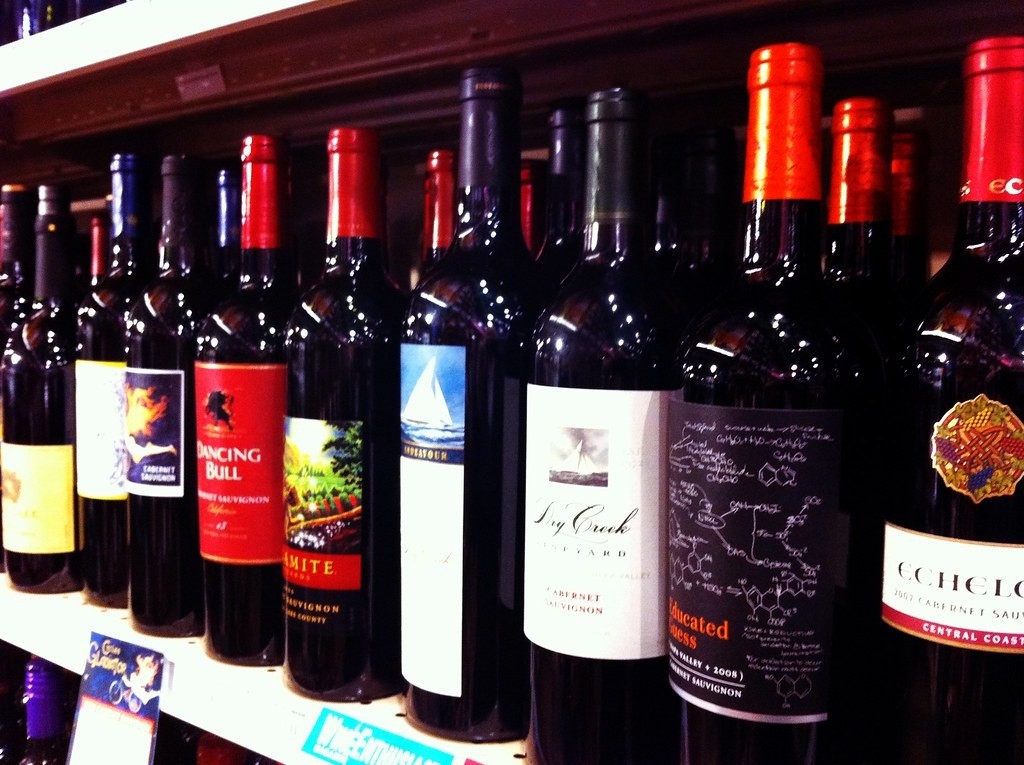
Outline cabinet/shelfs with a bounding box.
[0,0,1024,765]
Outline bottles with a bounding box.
[0,0,1024,765]
